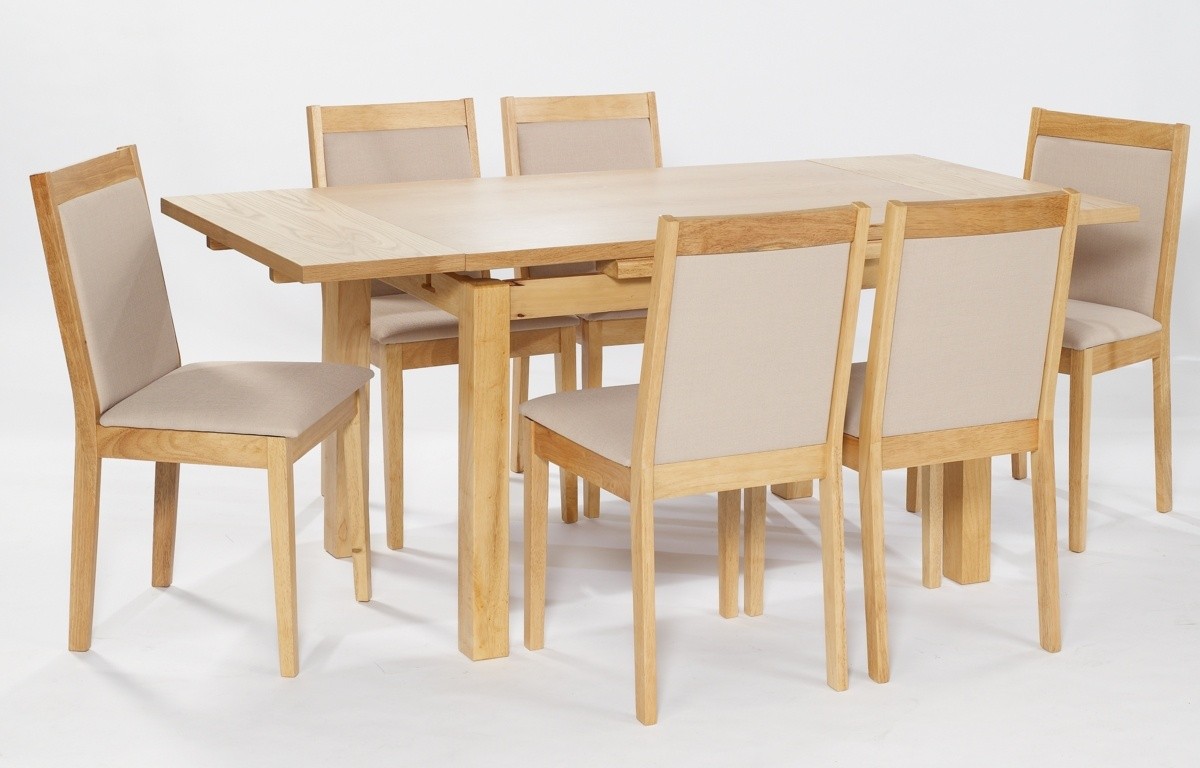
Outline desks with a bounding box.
[160,153,1142,662]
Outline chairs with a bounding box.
[905,107,1190,553]
[29,144,375,677]
[306,92,1080,726]
